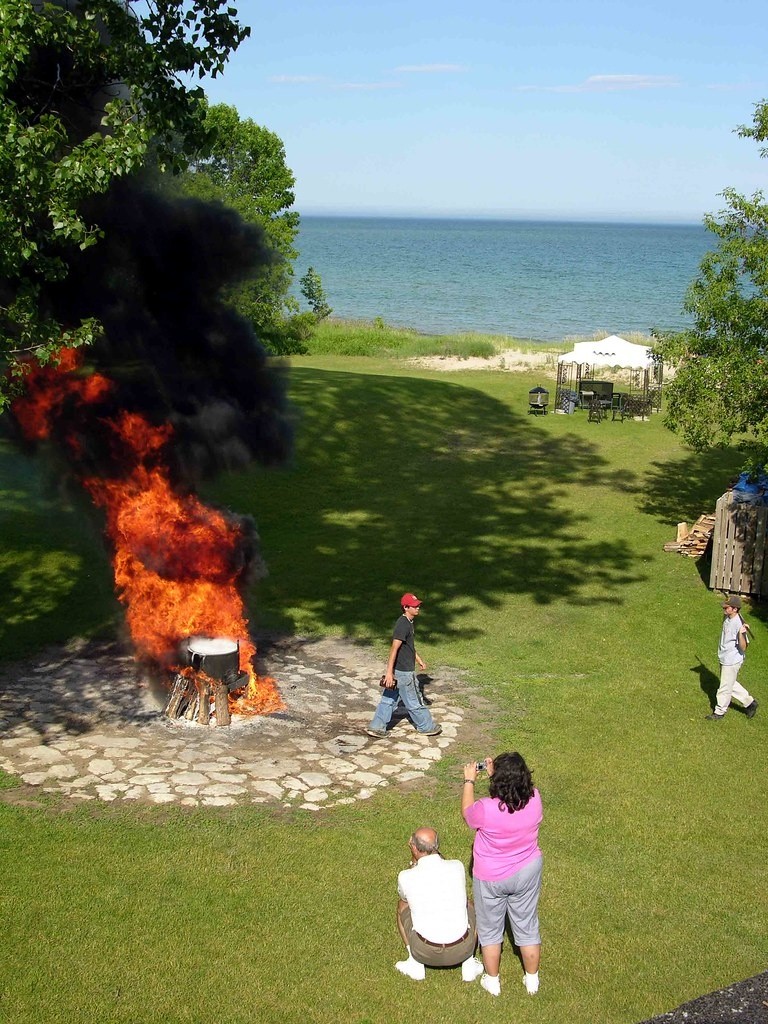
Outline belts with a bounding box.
[416,928,469,948]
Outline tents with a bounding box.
[558,335,661,420]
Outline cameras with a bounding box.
[477,763,487,770]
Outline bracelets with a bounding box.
[463,780,475,785]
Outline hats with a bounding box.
[721,596,741,608]
[401,593,422,606]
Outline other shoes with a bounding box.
[746,700,758,719]
[418,724,442,735]
[462,958,484,981]
[522,975,539,995]
[480,974,500,997]
[367,730,393,738]
[705,713,724,720]
[395,957,426,980]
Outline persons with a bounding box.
[395,828,484,982]
[705,595,758,722]
[363,593,443,738]
[462,753,545,997]
